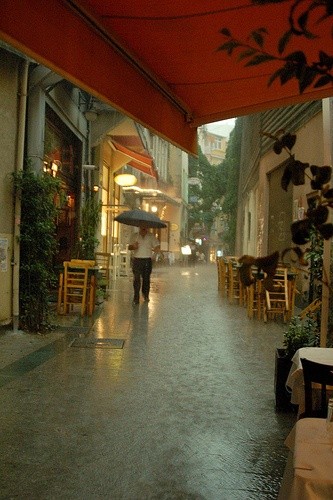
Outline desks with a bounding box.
[254,272,296,321]
[67,266,106,314]
[280,416,333,500]
[288,346,333,420]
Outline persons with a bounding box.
[128,220,160,306]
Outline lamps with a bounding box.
[79,101,100,121]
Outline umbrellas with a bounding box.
[113,208,168,255]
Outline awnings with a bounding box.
[107,137,160,183]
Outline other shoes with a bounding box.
[143,294,149,302]
[132,299,139,304]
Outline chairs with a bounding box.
[219,255,299,325]
[57,252,111,317]
[299,356,333,420]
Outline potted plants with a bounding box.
[274,310,321,417]
